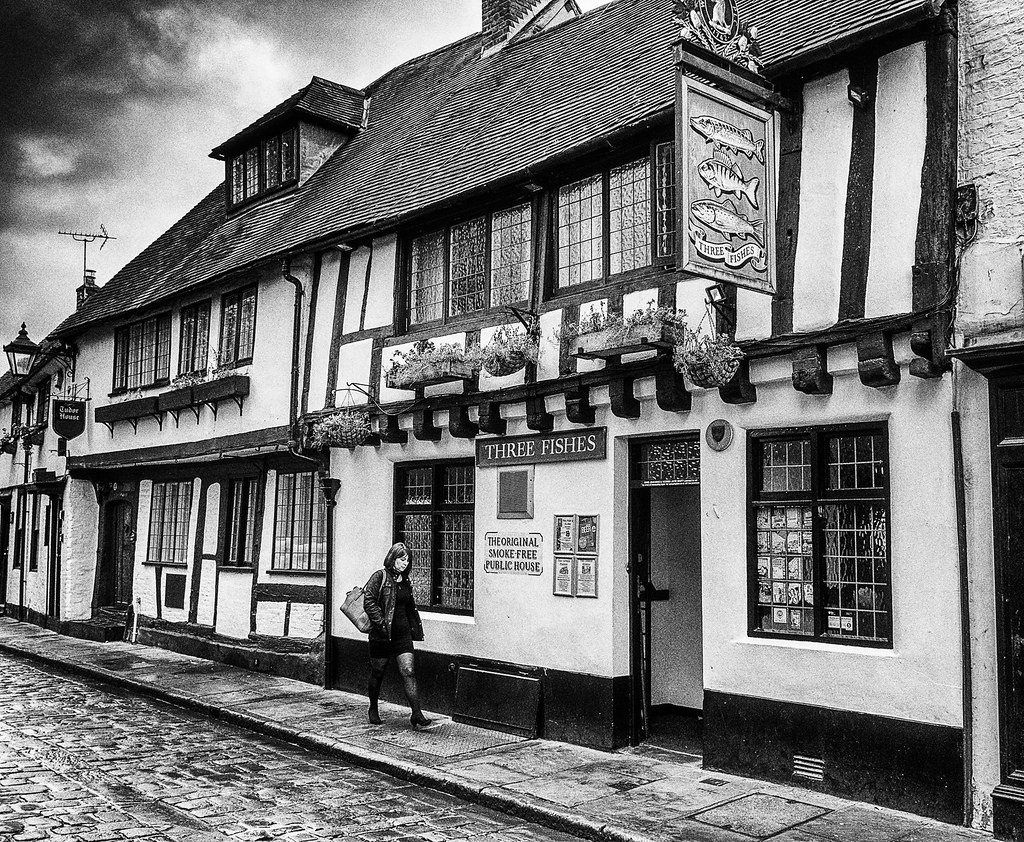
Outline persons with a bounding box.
[362,541,431,731]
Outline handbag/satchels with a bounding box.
[340,569,386,634]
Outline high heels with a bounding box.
[368,709,383,724]
[410,715,431,727]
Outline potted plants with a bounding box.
[93,388,165,422]
[309,410,373,451]
[0,427,17,455]
[194,347,250,403]
[545,297,689,359]
[382,338,473,390]
[12,420,45,445]
[158,372,203,411]
[472,320,546,378]
[669,325,748,391]
